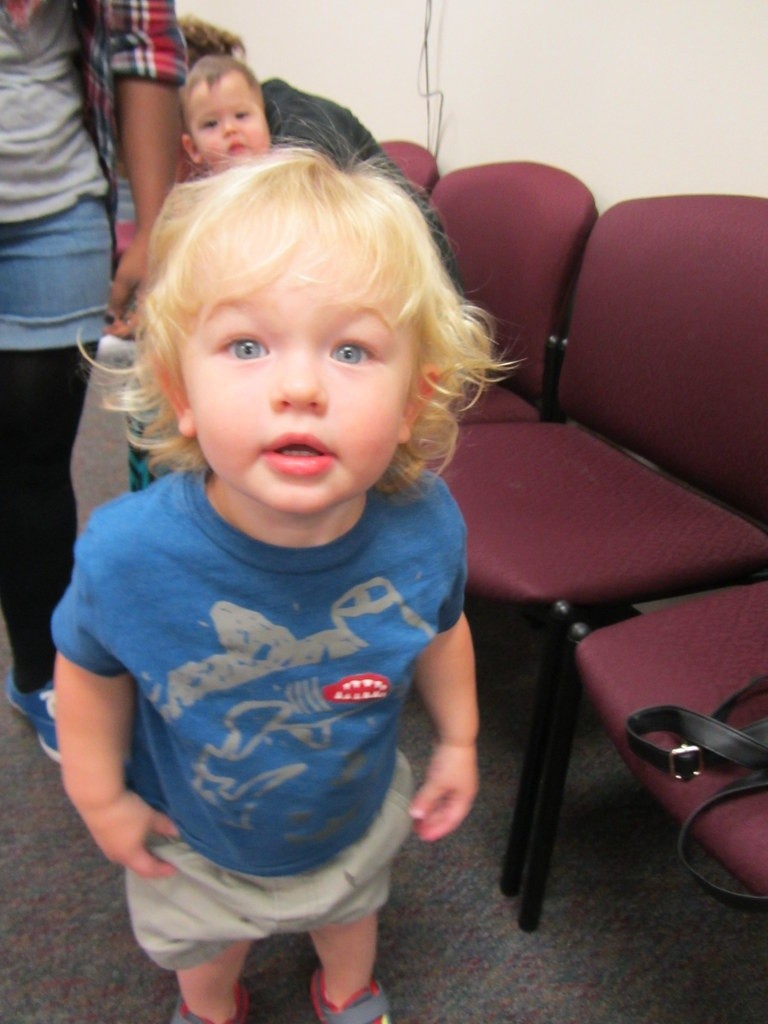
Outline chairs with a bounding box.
[374,138,601,424]
[419,191,768,894]
[516,581,768,931]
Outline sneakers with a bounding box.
[169,977,249,1024]
[311,966,390,1024]
[6,671,61,764]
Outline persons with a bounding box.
[181,56,270,172]
[0,0,187,763]
[175,18,459,280]
[52,149,507,1024]
